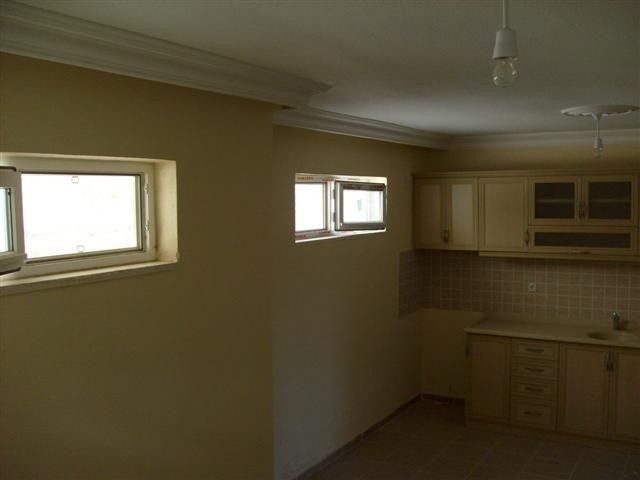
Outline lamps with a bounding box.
[561,104,640,159]
[492,0,520,88]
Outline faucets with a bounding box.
[610,311,621,329]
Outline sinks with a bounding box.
[587,328,639,344]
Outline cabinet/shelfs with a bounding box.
[557,343,640,445]
[478,170,529,257]
[413,171,478,251]
[529,167,640,261]
[508,338,559,432]
[464,335,510,424]
